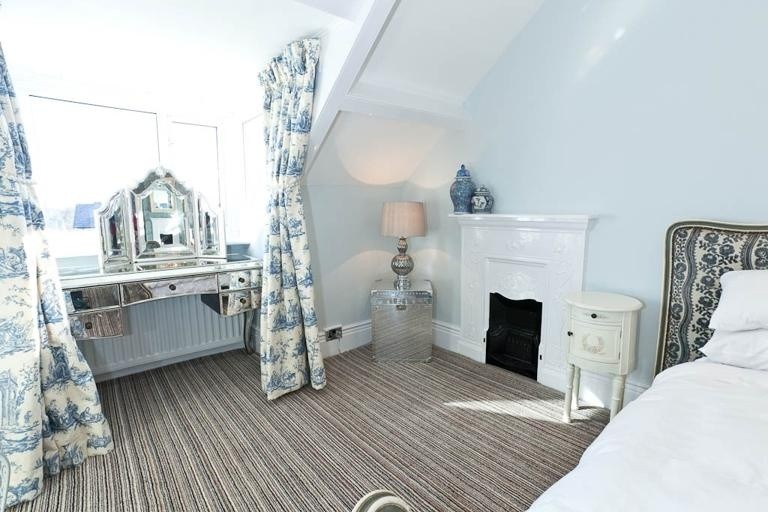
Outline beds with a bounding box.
[354,219,767,512]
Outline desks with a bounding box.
[369,278,435,363]
[46,251,267,373]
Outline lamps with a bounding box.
[378,198,428,290]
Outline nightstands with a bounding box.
[563,292,645,430]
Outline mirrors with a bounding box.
[96,164,228,266]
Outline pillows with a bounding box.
[707,267,768,334]
[698,325,767,375]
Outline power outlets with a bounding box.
[324,324,343,341]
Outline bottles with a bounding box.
[449,164,476,215]
[472,186,493,214]
